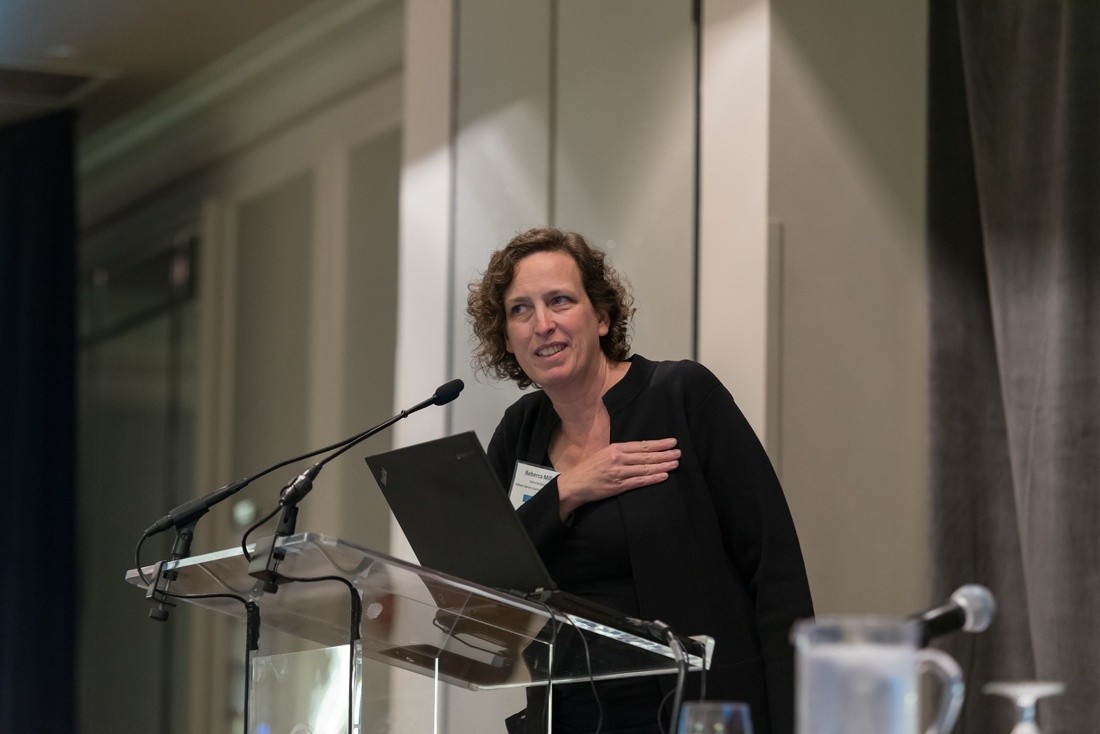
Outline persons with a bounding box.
[465,229,815,734]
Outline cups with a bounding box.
[676,702,752,734]
[791,613,967,734]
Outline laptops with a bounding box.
[365,430,706,659]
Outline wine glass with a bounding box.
[980,679,1068,734]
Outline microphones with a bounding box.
[907,582,998,652]
[146,379,465,539]
